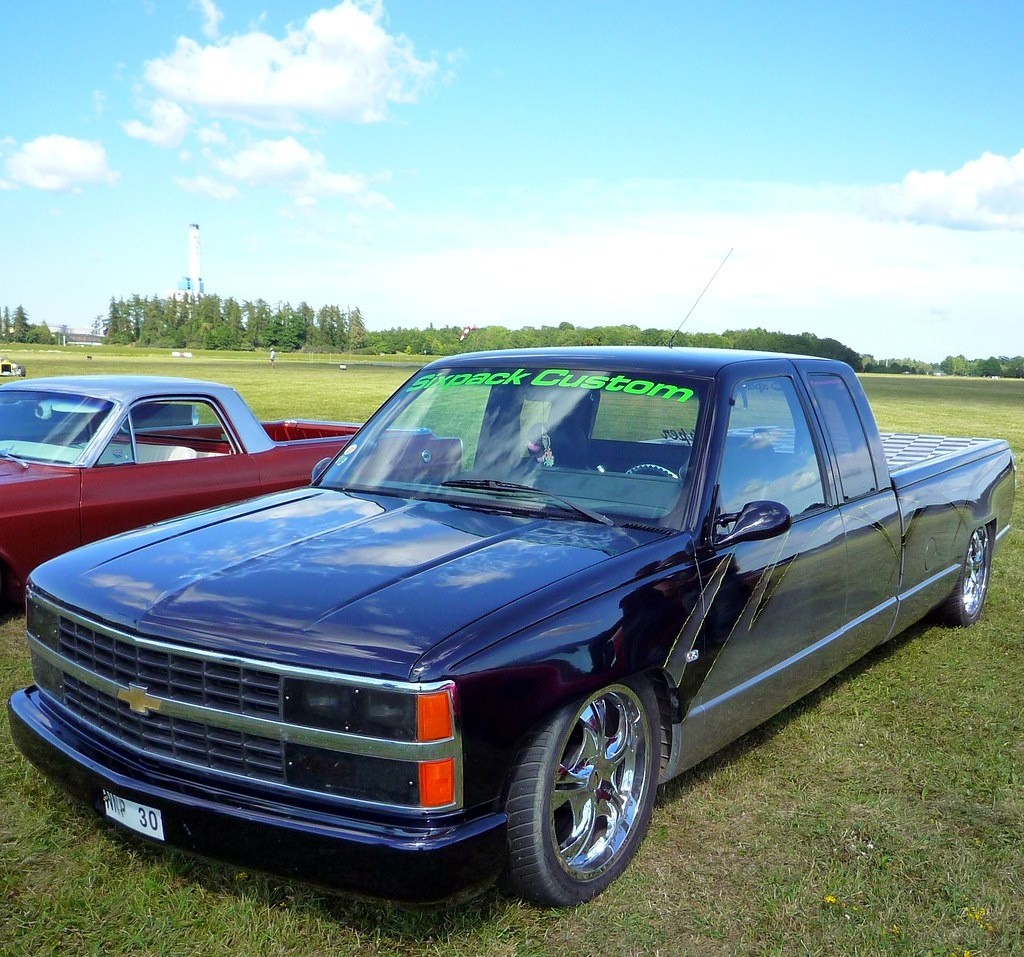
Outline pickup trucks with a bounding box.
[7,346,1015,911]
[0,375,462,613]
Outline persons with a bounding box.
[270,348,276,368]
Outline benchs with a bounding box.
[568,437,789,479]
[79,443,198,465]
